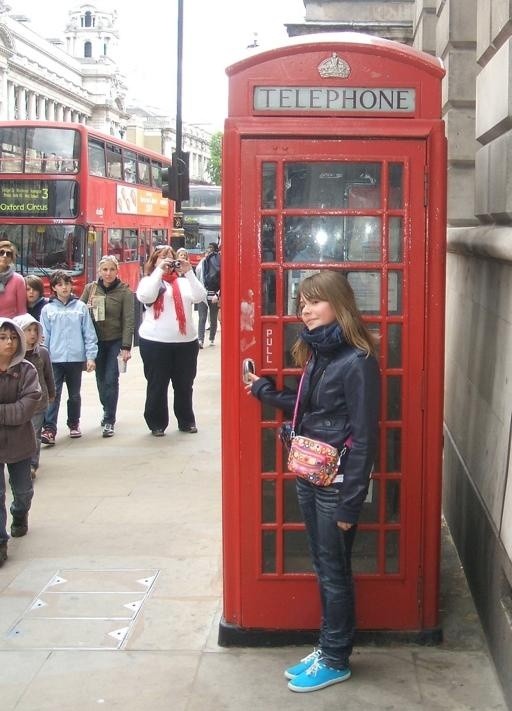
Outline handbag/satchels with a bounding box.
[286,435,340,487]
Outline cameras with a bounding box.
[170,259,181,266]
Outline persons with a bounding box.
[176,241,222,348]
[0,240,27,319]
[243,270,382,693]
[39,269,99,444]
[135,244,207,436]
[78,255,135,437]
[13,312,56,480]
[0,316,43,566]
[24,274,53,322]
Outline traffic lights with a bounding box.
[158,160,172,202]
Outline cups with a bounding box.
[117,353,127,374]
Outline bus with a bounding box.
[1,121,174,300]
[181,183,221,271]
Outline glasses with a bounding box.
[0,249,12,258]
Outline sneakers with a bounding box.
[210,341,216,345]
[180,425,197,433]
[40,415,113,444]
[288,655,351,692]
[285,647,322,680]
[11,513,29,537]
[153,429,164,436]
[0,547,7,565]
[198,339,203,349]
